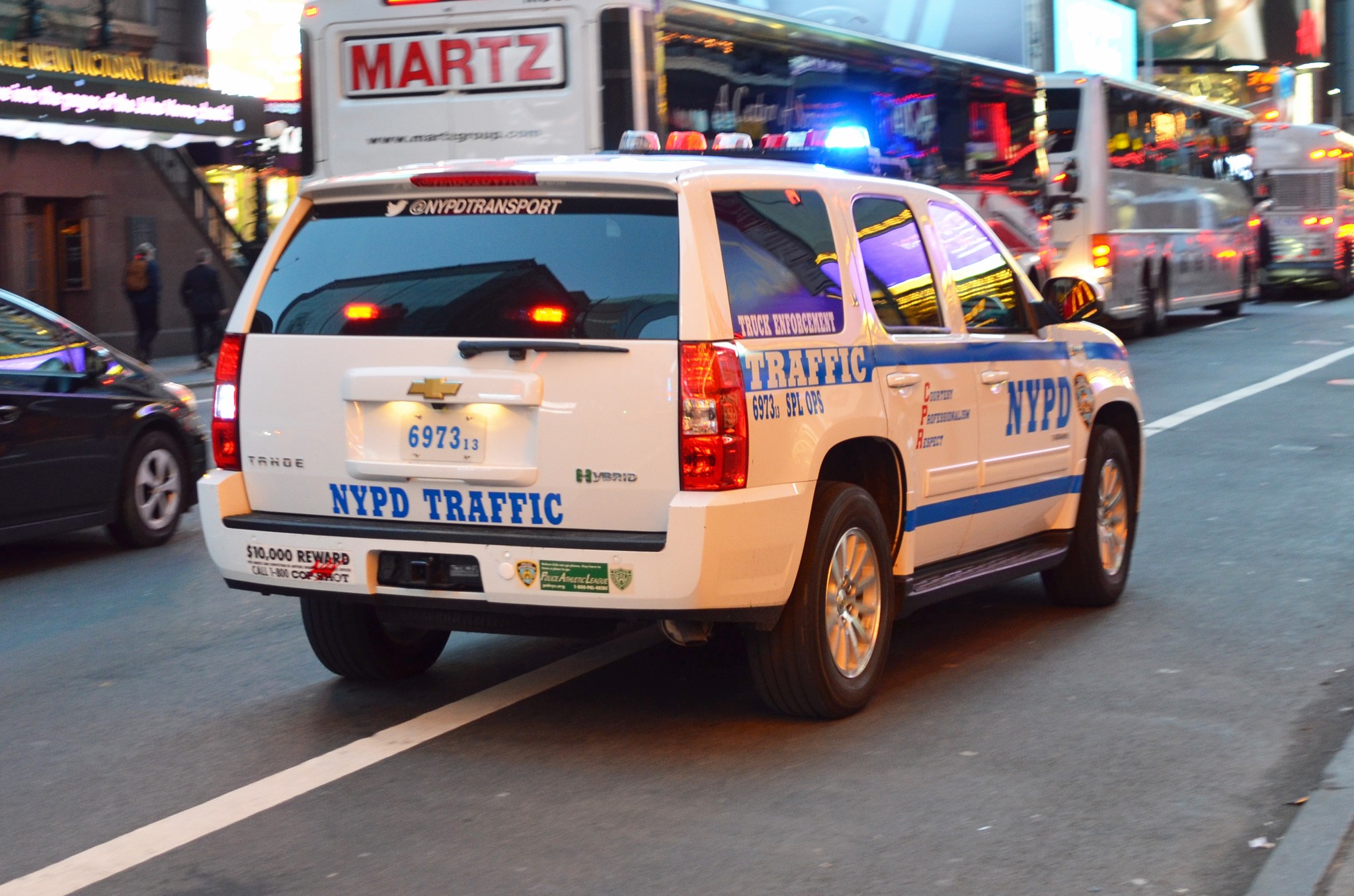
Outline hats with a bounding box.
[137,243,156,253]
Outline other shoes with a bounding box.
[198,347,214,366]
[137,350,148,364]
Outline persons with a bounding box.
[178,249,230,370]
[1122,0,1255,59]
[121,243,164,368]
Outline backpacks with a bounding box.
[126,258,149,292]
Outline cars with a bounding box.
[0,288,207,549]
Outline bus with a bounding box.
[1041,68,1267,332]
[1249,125,1354,300]
[297,1,1059,290]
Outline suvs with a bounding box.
[196,131,1144,722]
